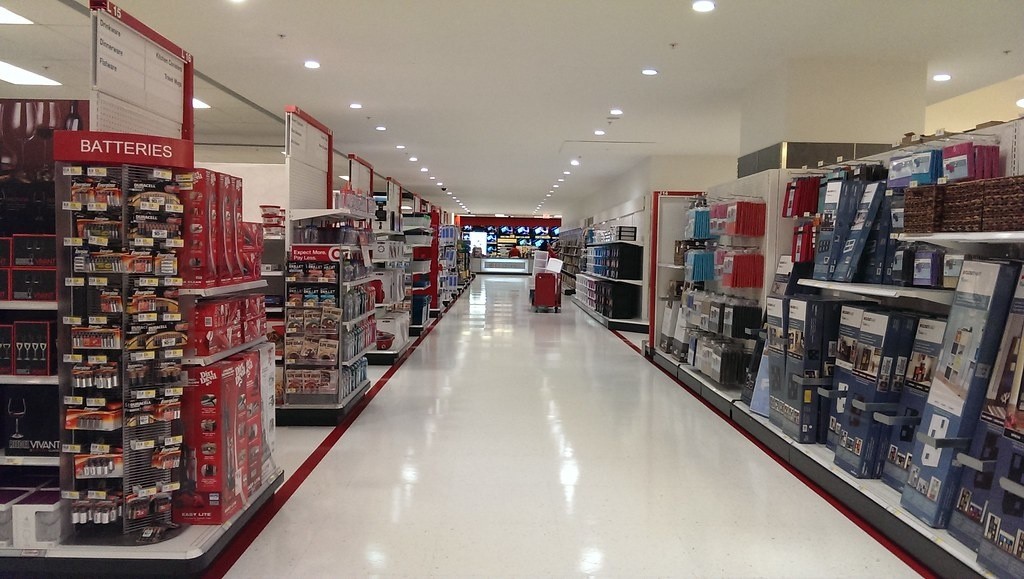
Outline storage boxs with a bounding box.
[1,233,61,456]
[741,142,1024,579]
[172,167,278,525]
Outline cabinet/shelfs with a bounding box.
[194,156,463,425]
[1,130,70,553]
[558,227,649,333]
[650,119,1024,579]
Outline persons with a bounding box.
[509,243,521,258]
[546,242,558,259]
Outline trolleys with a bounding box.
[534,274,560,312]
